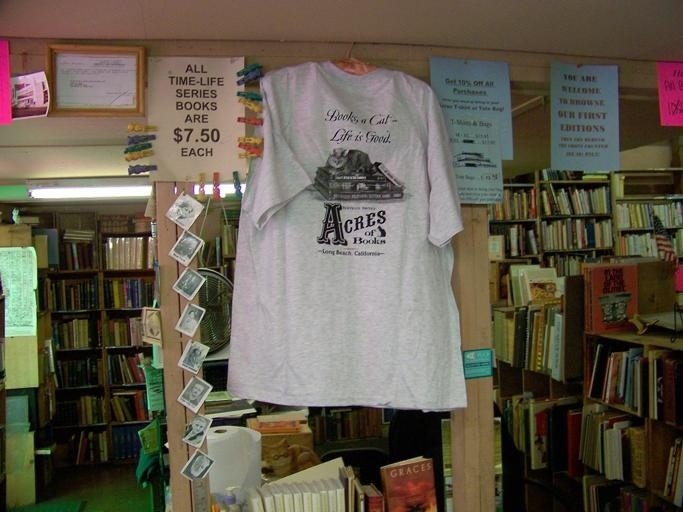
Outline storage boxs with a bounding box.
[0,218,56,510]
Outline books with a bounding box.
[217,202,241,283]
[201,389,251,415]
[19,212,159,490]
[242,406,487,512]
[487,167,683,512]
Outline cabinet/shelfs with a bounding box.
[22,166,683,512]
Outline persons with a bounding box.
[162,189,216,480]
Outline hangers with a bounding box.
[335,41,379,74]
